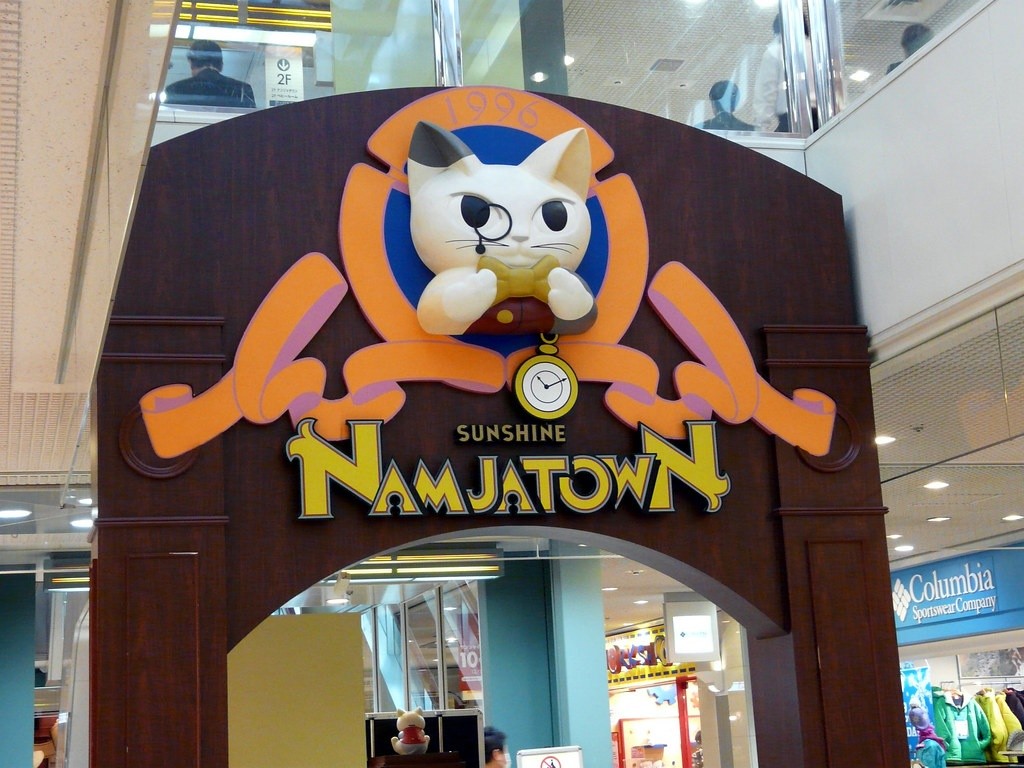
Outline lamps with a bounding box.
[322,549,505,584]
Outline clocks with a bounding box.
[514,355,580,419]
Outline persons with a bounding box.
[164,39,257,108]
[482,726,513,768]
[692,80,755,132]
[754,11,819,134]
[885,23,935,76]
[908,703,946,768]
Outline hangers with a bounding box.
[944,682,1016,696]
